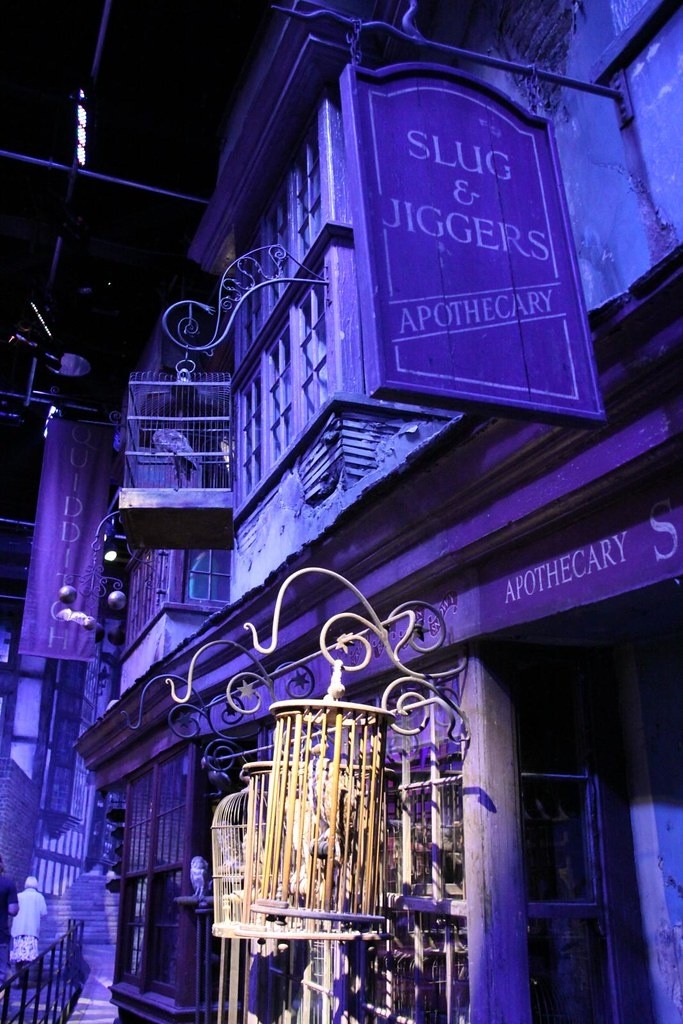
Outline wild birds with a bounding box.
[149,426,198,481]
[189,855,212,899]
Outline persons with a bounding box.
[0,857,19,1000]
[11,876,47,989]
